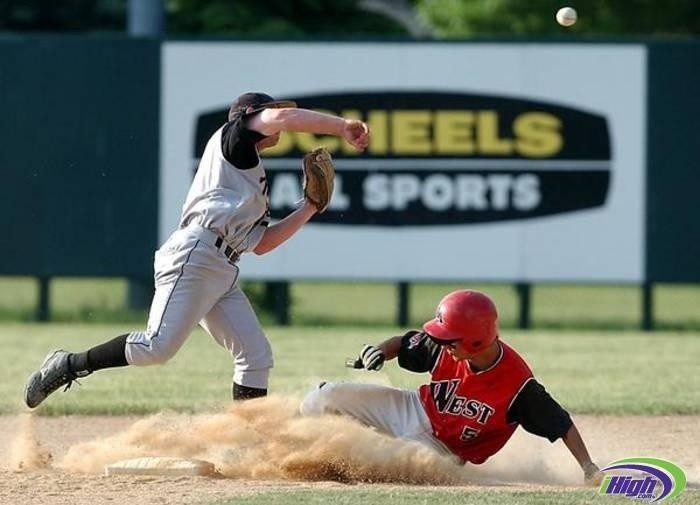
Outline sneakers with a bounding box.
[23,345,82,411]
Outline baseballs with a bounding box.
[556,6,577,27]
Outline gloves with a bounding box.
[341,342,386,376]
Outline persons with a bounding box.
[23,90,373,412]
[299,288,607,488]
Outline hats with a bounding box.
[228,90,300,113]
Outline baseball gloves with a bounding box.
[303,148,335,215]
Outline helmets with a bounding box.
[421,288,501,357]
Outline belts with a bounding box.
[185,220,243,267]
